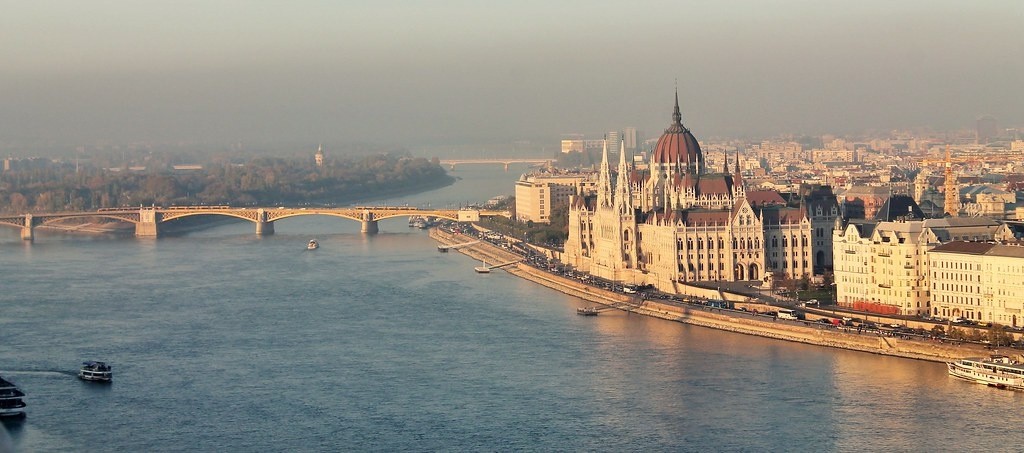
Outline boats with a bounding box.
[77,361,113,385]
[0,377,27,420]
[308,239,319,250]
[945,354,1024,389]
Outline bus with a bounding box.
[778,309,798,320]
